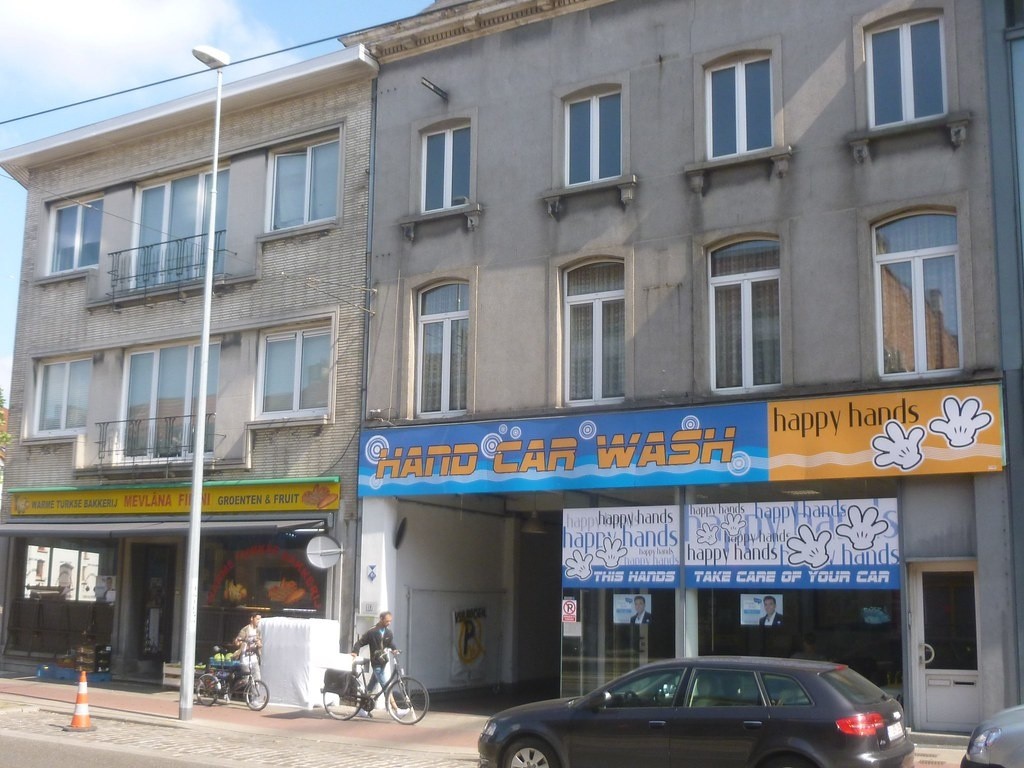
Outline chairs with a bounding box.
[691,676,723,708]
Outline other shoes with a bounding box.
[251,701,260,706]
[225,693,231,701]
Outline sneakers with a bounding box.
[396,708,410,718]
[357,707,368,716]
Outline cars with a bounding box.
[477,656,916,768]
[960,704,1024,768]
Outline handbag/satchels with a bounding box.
[374,649,389,665]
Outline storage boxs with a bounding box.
[36,644,113,683]
[162,656,242,691]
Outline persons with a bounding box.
[759,596,783,626]
[630,597,652,624]
[103,577,116,602]
[235,612,263,707]
[352,612,410,720]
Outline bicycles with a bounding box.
[321,651,429,724]
[195,639,269,710]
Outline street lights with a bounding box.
[177,45,228,719]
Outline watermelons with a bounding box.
[214,652,232,662]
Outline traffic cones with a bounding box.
[63,671,97,732]
